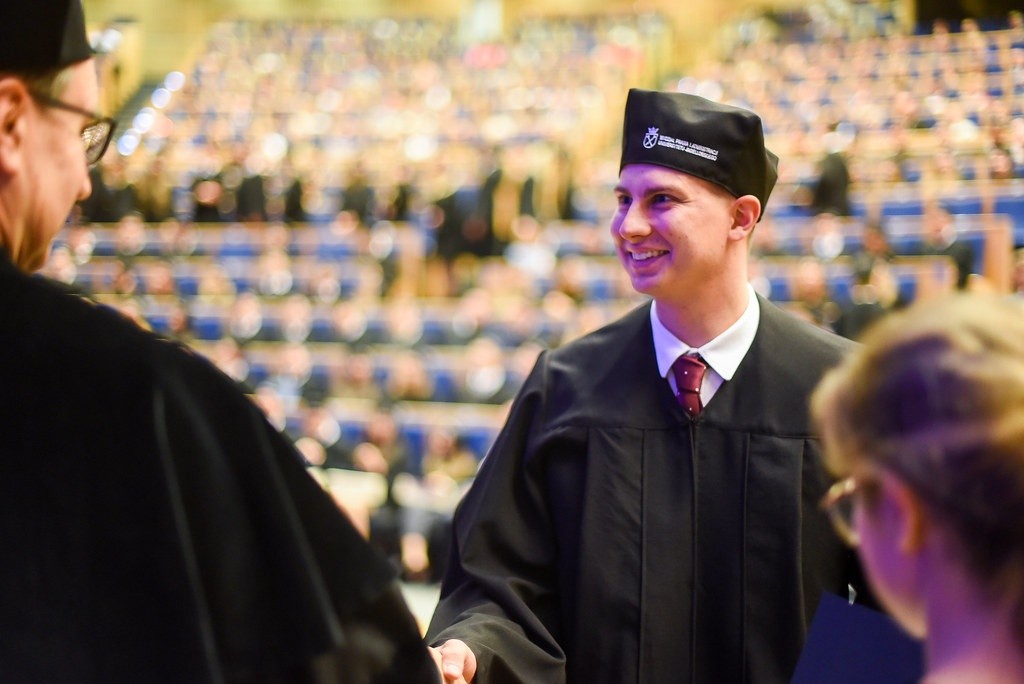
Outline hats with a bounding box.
[0,1,95,79]
[619,88,780,223]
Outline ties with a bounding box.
[671,353,709,415]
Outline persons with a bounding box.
[33,0,1024,591]
[425,87,873,684]
[809,291,1024,684]
[0,1,446,684]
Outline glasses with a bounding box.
[22,87,116,169]
[820,474,877,547]
